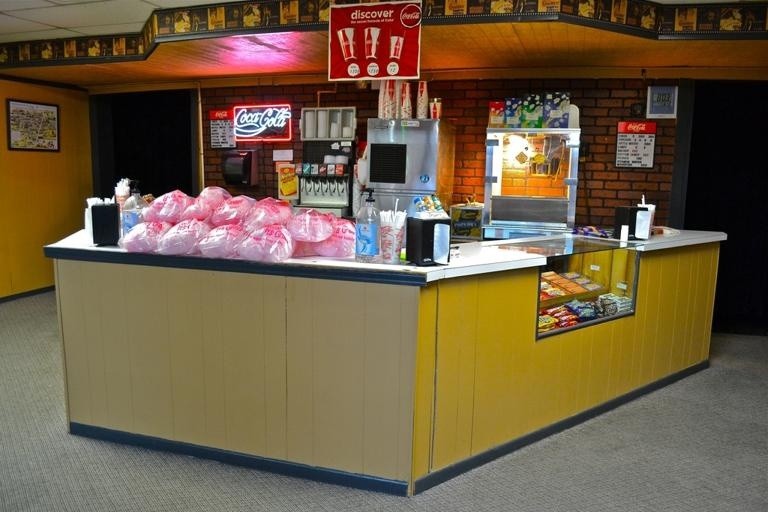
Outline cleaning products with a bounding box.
[354,187,381,262]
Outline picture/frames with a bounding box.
[4,94,61,155]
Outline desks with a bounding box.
[45,209,727,495]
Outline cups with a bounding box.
[377,79,444,122]
[389,36,403,59]
[364,27,381,60]
[115,187,130,212]
[121,209,139,238]
[380,222,405,265]
[636,204,656,237]
[337,26,359,61]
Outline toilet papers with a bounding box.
[635,211,651,240]
